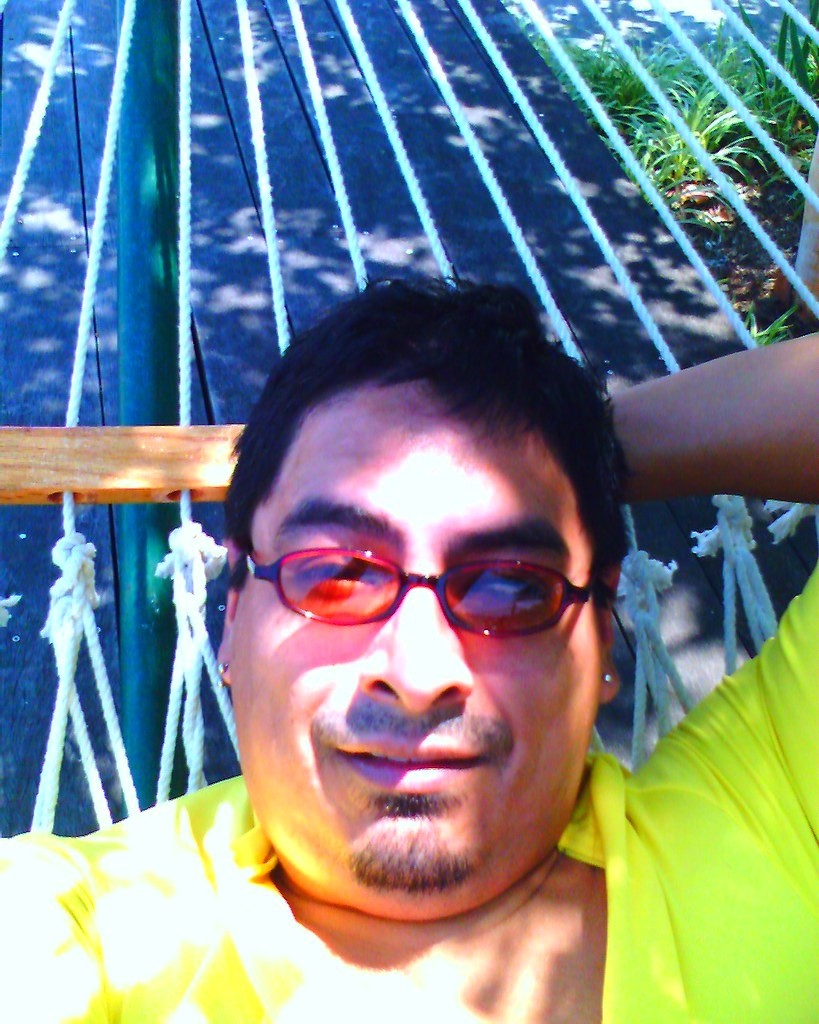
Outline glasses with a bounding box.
[246,548,603,638]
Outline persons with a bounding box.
[0,275,819,1024]
